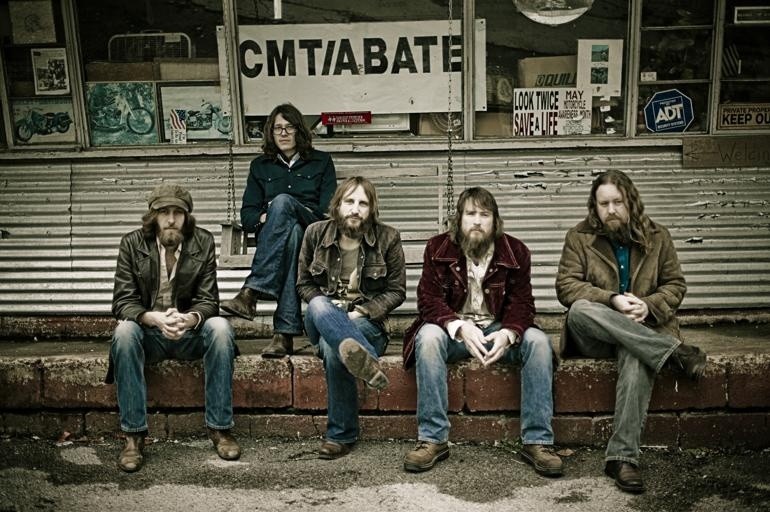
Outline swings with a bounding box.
[217,1,459,271]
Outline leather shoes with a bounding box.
[665,341,706,379]
[208,426,241,460]
[604,457,645,494]
[338,338,389,389]
[317,437,351,460]
[119,434,146,472]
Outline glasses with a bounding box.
[273,124,296,135]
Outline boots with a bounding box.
[262,335,297,360]
[220,287,260,321]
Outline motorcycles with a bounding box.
[168,99,232,135]
[89,89,154,136]
[14,107,73,142]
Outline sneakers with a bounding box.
[403,439,451,472]
[520,443,563,476]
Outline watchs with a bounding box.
[399,187,564,478]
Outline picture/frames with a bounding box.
[155,80,234,142]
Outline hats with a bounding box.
[148,183,193,213]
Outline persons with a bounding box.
[101,181,244,472]
[554,168,710,492]
[218,101,341,361]
[293,175,407,460]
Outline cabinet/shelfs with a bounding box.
[631,0,770,136]
